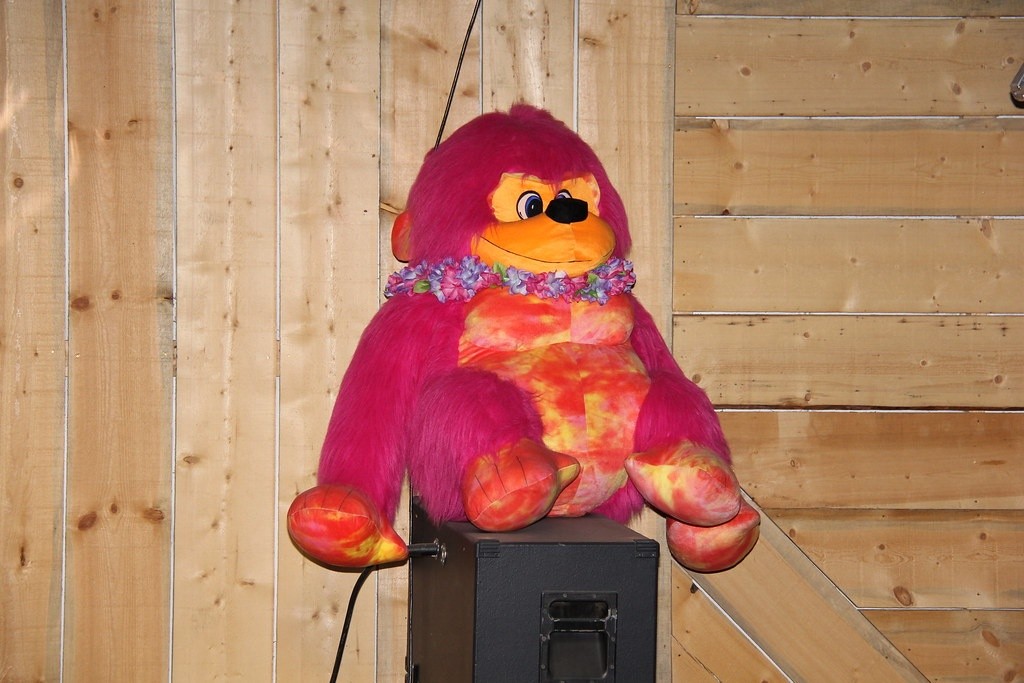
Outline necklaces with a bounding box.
[384,254,637,305]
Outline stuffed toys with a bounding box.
[287,101,760,574]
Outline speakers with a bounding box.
[409,495,661,683]
